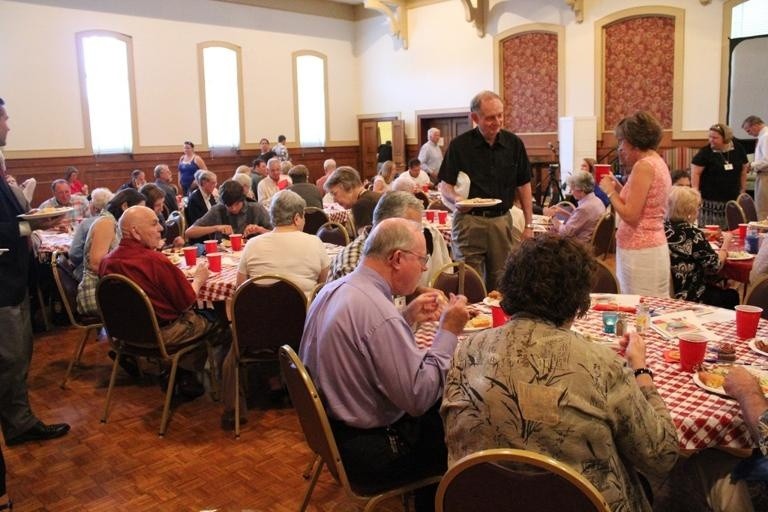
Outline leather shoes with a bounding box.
[5,419,71,447]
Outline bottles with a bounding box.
[641,307,651,333]
[616,313,628,337]
[636,299,644,332]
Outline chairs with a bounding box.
[316,222,349,247]
[229,275,308,439]
[555,201,577,215]
[737,192,758,223]
[591,212,615,262]
[347,210,358,238]
[94,275,218,437]
[48,247,103,390]
[592,258,619,293]
[435,448,610,512]
[167,211,185,241]
[741,273,768,319]
[725,200,745,230]
[302,206,327,236]
[432,261,487,307]
[275,343,441,512]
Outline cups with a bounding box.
[177,196,182,203]
[676,333,709,372]
[205,254,222,272]
[439,211,448,224]
[229,234,242,251]
[489,304,509,328]
[738,224,747,239]
[426,212,434,223]
[422,185,428,193]
[204,241,218,253]
[705,225,720,241]
[183,247,198,266]
[594,165,611,186]
[735,304,762,340]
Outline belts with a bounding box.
[467,208,508,218]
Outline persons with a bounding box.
[187,172,217,243]
[252,160,266,196]
[140,183,179,246]
[185,181,274,322]
[76,188,149,378]
[316,159,336,198]
[188,169,219,197]
[65,166,88,194]
[256,159,293,212]
[219,165,251,204]
[61,188,114,311]
[543,170,605,252]
[691,123,749,228]
[437,91,534,298]
[299,216,471,512]
[153,164,184,220]
[39,178,91,324]
[673,364,768,511]
[373,161,396,194]
[417,126,444,186]
[97,206,214,407]
[236,188,331,403]
[286,164,323,212]
[234,174,255,200]
[272,135,289,162]
[392,159,430,193]
[0,97,70,448]
[375,141,393,173]
[571,158,608,207]
[252,139,276,169]
[599,109,672,301]
[744,234,768,302]
[663,186,739,308]
[4,174,46,334]
[117,170,147,195]
[742,115,768,234]
[663,170,700,225]
[322,166,381,235]
[280,161,293,176]
[178,141,207,197]
[326,191,425,287]
[439,233,679,512]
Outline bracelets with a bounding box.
[525,223,534,228]
[633,366,651,376]
[606,191,615,198]
[719,247,730,257]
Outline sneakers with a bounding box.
[221,415,249,430]
[106,349,140,375]
[166,384,205,406]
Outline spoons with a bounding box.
[220,243,233,253]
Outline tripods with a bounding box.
[535,171,564,206]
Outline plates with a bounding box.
[727,251,754,260]
[483,297,502,308]
[161,249,184,256]
[17,208,74,218]
[435,325,490,333]
[748,337,767,355]
[692,366,762,396]
[532,219,564,225]
[456,199,502,206]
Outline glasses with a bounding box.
[331,182,344,196]
[393,249,430,266]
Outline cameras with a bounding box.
[547,160,559,168]
